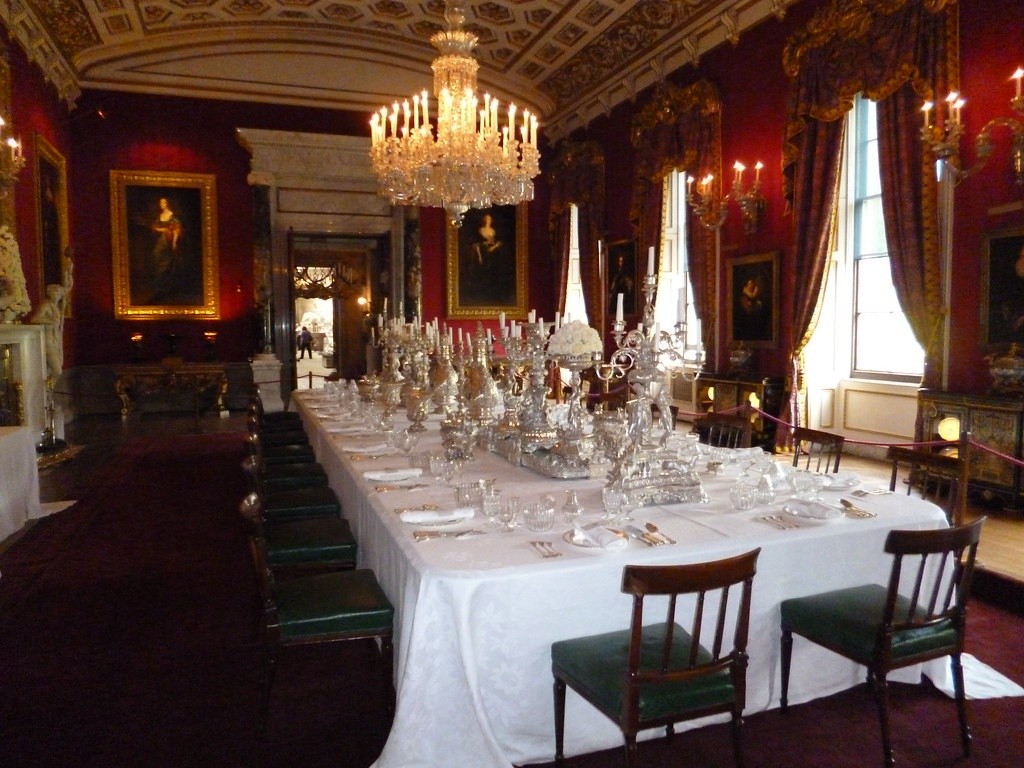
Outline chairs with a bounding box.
[240,382,395,768]
[552,403,989,768]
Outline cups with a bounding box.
[729,484,759,510]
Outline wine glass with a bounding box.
[322,378,479,488]
[456,478,637,533]
[684,432,815,511]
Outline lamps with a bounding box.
[129,332,143,362]
[685,158,767,237]
[367,0,543,228]
[204,330,220,363]
[919,65,1024,187]
[0,118,26,185]
[293,237,368,301]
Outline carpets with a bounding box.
[0,432,1023,767]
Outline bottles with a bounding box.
[758,476,774,504]
[560,488,584,525]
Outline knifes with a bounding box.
[414,530,484,537]
[628,525,660,546]
[840,498,878,516]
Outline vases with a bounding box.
[1,308,19,322]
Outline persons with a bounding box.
[296,324,314,362]
[30,284,64,388]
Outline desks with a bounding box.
[294,389,1024,768]
[108,360,250,419]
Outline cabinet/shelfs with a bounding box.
[693,371,783,449]
[910,386,1024,515]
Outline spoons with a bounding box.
[645,523,677,544]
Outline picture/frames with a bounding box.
[978,226,1024,357]
[725,249,780,352]
[109,169,221,320]
[34,133,76,319]
[444,201,530,322]
[604,237,637,317]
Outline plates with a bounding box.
[346,445,382,454]
[330,427,363,435]
[823,477,862,491]
[782,502,846,525]
[308,405,339,409]
[410,516,465,526]
[316,412,349,418]
[367,477,410,482]
[562,526,629,552]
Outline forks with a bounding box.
[851,489,892,497]
[417,529,473,543]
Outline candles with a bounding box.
[371,246,703,356]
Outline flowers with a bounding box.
[0,224,31,319]
[545,320,602,357]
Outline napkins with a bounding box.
[302,396,627,552]
